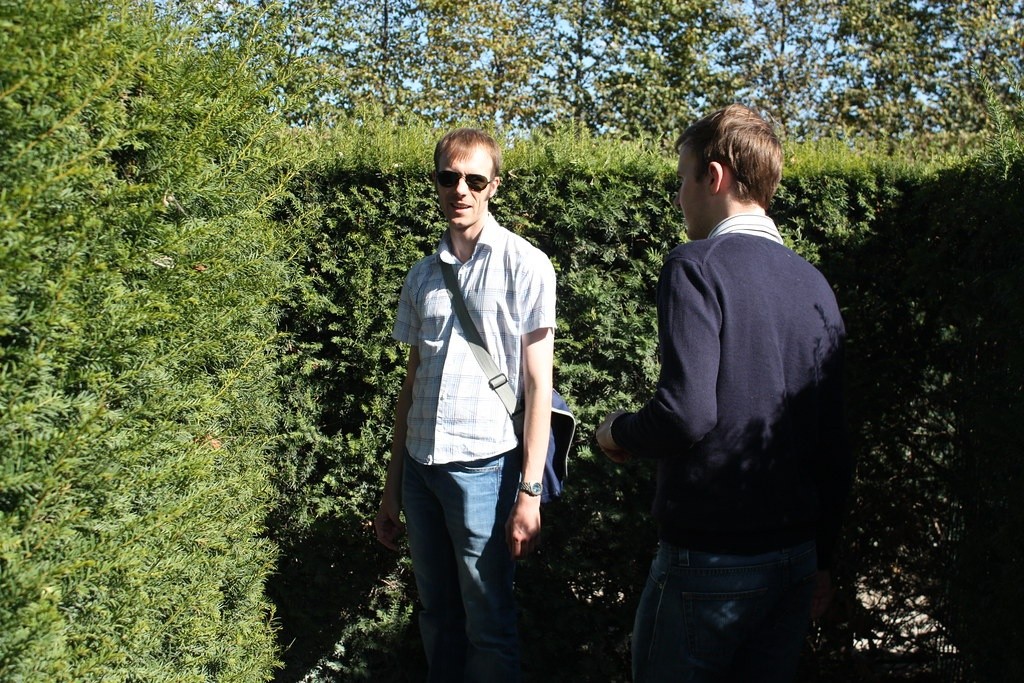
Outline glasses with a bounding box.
[435,169,493,191]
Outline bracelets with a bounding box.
[384,481,401,505]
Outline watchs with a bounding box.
[519,481,543,496]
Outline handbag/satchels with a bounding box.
[522,387,576,505]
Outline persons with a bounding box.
[596,104,852,683]
[375,128,557,683]
